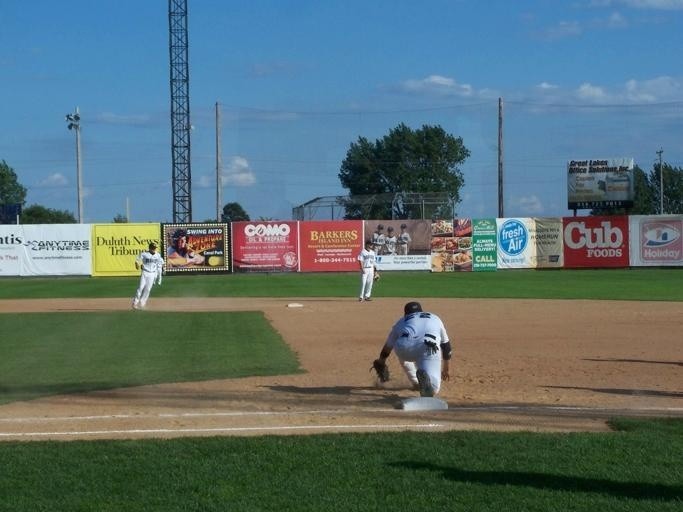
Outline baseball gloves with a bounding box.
[373,274,381,282]
[374,359,389,382]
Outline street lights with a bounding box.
[654,147,666,213]
[63,104,84,223]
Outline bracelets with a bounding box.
[380,351,389,359]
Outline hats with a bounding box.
[149,243,158,249]
[400,224,406,228]
[387,226,393,232]
[365,240,376,246]
[378,224,384,230]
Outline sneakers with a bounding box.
[416,368,434,397]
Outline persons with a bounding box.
[358,240,379,302]
[373,301,452,396]
[167,231,205,266]
[369,223,410,255]
[132,243,163,309]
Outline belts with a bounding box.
[401,333,436,339]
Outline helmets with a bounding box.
[404,302,422,315]
